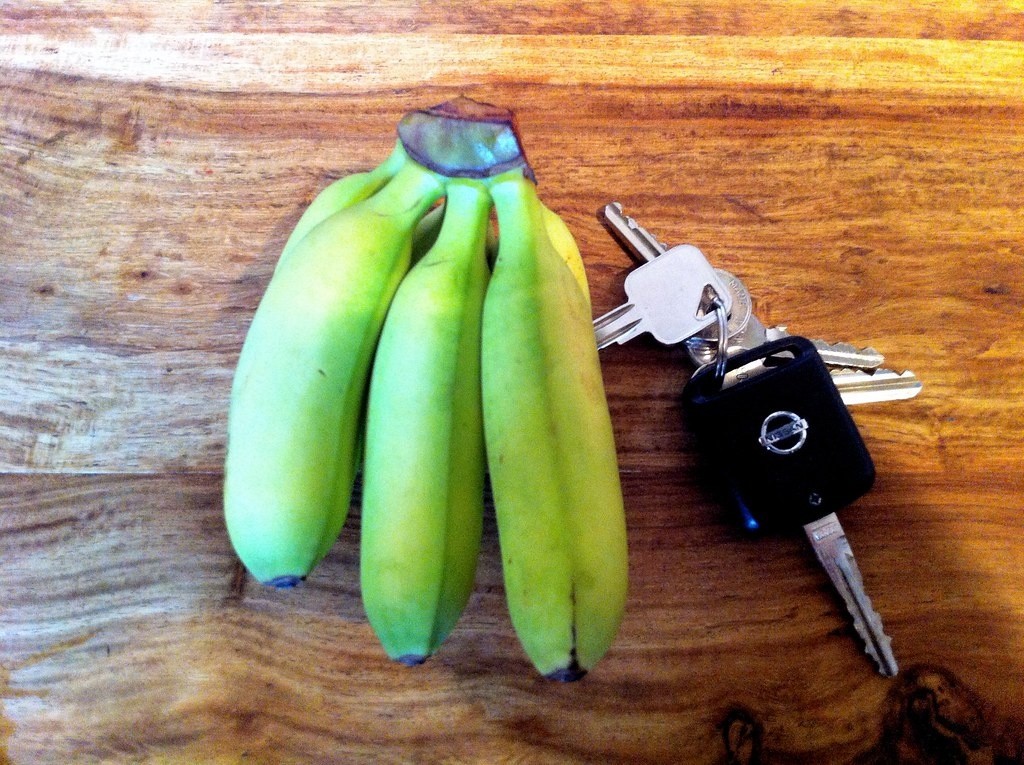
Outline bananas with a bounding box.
[219,95,631,685]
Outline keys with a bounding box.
[591,202,923,405]
[682,336,901,680]
